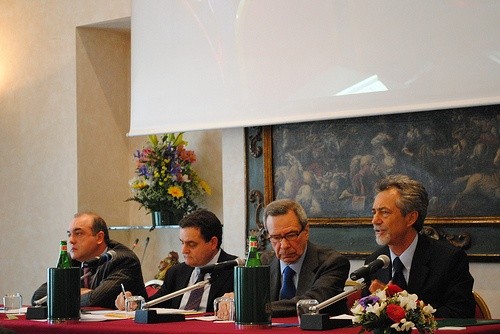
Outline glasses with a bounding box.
[268,224,305,243]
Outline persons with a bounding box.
[361,177,476,319]
[31,210,149,310]
[114,210,240,311]
[216,199,350,321]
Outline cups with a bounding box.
[213,297,235,320]
[47,267,81,324]
[2,293,22,311]
[233,265,273,329]
[125,296,145,316]
[295,299,320,326]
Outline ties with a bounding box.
[392,256,408,291]
[184,268,206,310]
[280,266,296,299]
[83,267,89,289]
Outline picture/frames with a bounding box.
[262,104,500,226]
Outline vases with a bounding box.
[151,210,180,225]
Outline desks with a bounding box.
[0,305,500,334]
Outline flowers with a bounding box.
[350,280,438,334]
[123,133,212,219]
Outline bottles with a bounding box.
[56,240,71,270]
[244,235,262,268]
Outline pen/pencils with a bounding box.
[0,308,7,310]
[121,284,126,300]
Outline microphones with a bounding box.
[140,237,149,264]
[83,249,117,269]
[350,254,390,281]
[197,257,246,276]
[131,238,139,250]
